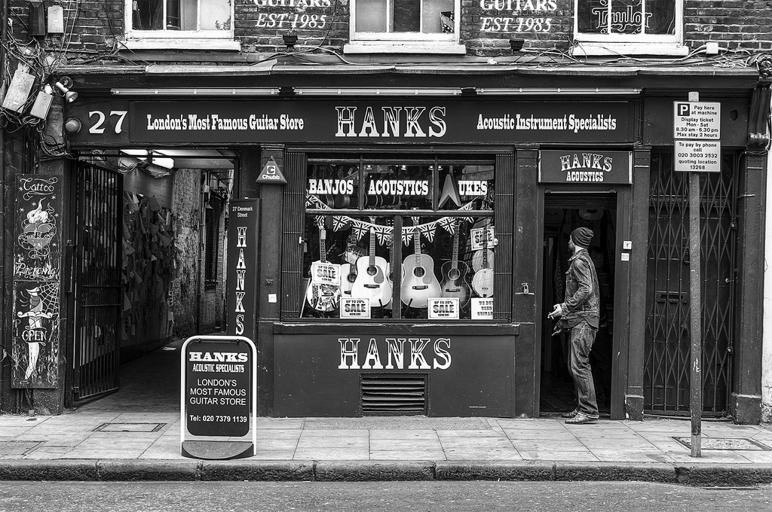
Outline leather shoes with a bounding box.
[565,412,599,424]
[562,409,578,418]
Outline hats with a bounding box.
[571,227,594,248]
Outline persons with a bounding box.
[547,227,600,424]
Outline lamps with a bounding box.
[507,34,526,52]
[282,29,299,49]
[53,80,81,104]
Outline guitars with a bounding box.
[306,199,495,312]
[307,163,399,208]
[437,165,462,209]
